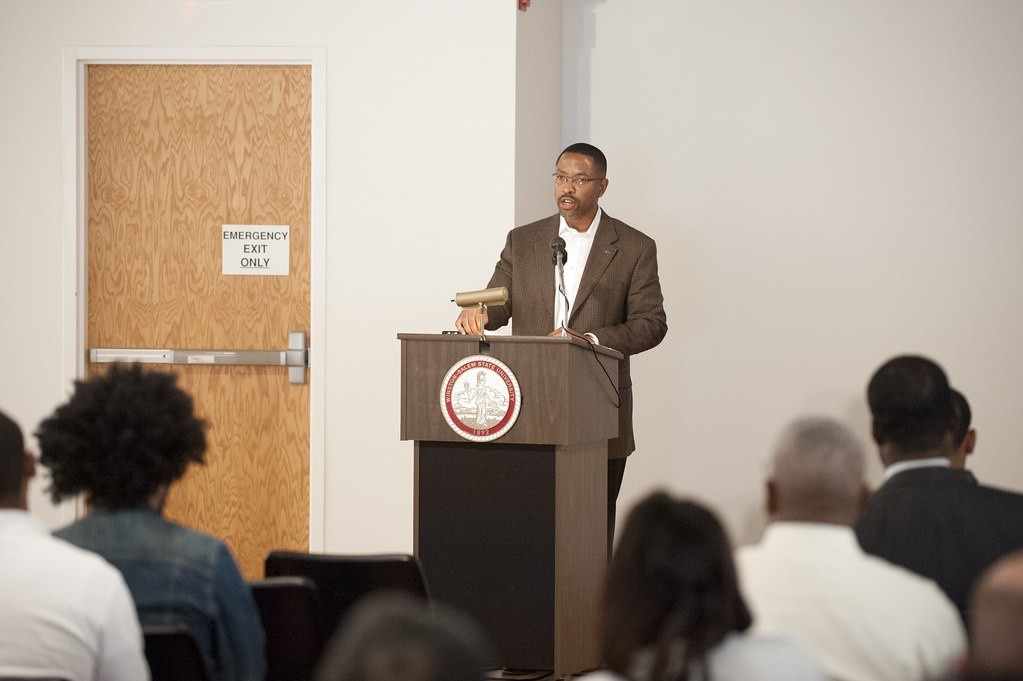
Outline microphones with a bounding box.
[550,237,566,251]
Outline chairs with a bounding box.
[244,577,331,681]
[142,623,207,681]
[263,548,430,629]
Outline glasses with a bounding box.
[552,174,603,186]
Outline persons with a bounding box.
[949,387,1023,511]
[706,416,970,681]
[951,552,1023,681]
[856,355,1023,632]
[455,143,668,561]
[323,587,503,681]
[32,359,264,681]
[1,410,153,681]
[577,491,752,680]
